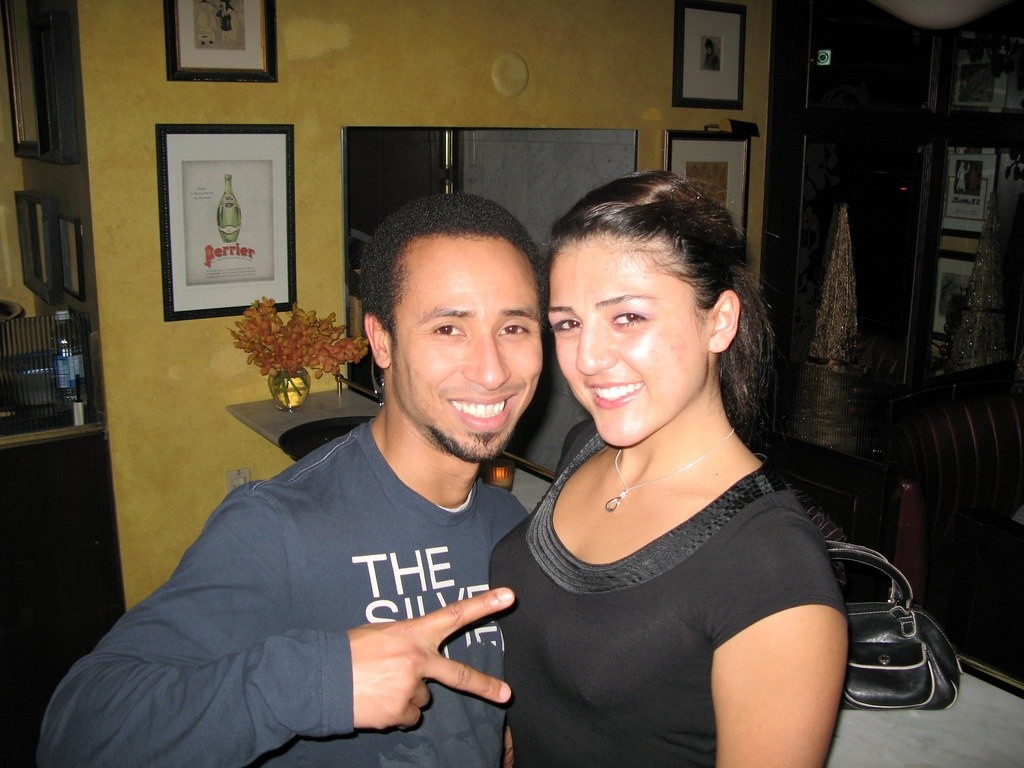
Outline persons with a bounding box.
[489,168,849,768]
[37,191,546,768]
[956,162,970,192]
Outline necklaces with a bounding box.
[604,427,735,514]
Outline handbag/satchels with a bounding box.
[824,540,962,710]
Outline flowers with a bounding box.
[227,297,370,377]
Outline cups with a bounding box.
[487,459,515,492]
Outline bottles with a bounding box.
[218,174,241,243]
[53,310,87,412]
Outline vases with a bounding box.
[269,362,311,410]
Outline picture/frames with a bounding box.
[157,122,296,323]
[671,1,747,110]
[1,1,84,164]
[16,188,87,305]
[161,0,277,83]
[662,129,753,246]
[914,29,1024,376]
